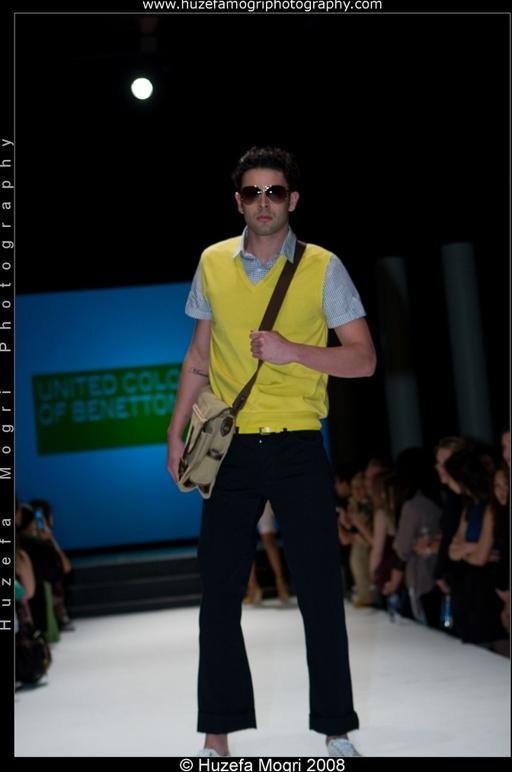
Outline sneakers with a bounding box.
[193,747,232,757]
[325,737,365,758]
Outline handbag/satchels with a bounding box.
[175,383,238,501]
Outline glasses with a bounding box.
[239,184,293,206]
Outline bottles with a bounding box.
[369,574,383,611]
[440,593,453,630]
[421,527,434,554]
[388,590,400,625]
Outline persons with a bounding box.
[246,431,512,658]
[13,496,80,690]
[165,147,378,756]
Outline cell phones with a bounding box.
[34,507,45,539]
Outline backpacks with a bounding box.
[14,615,53,687]
